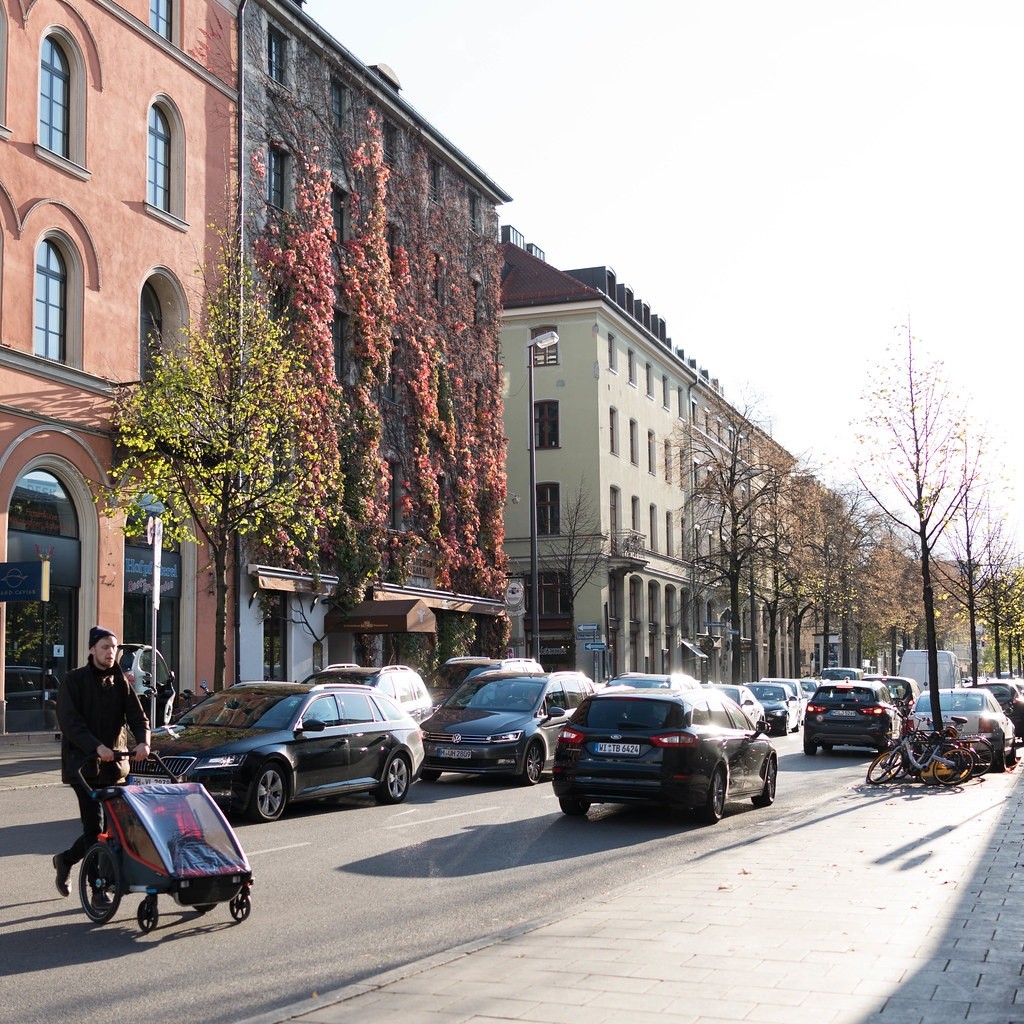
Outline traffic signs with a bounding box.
[575,634,600,640]
[582,641,605,652]
[576,622,597,633]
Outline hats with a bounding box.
[86,625,114,649]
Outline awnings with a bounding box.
[323,599,436,634]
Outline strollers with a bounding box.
[76,750,255,935]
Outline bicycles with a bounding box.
[866,700,994,788]
[167,680,215,724]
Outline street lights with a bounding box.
[527,332,561,677]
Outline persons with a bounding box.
[53,626,152,914]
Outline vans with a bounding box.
[896,649,961,695]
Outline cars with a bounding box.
[706,684,766,733]
[758,678,809,726]
[736,682,802,735]
[790,667,1024,771]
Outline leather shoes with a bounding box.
[51,851,72,896]
[89,893,113,911]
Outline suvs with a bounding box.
[424,656,544,709]
[298,662,433,733]
[4,665,61,711]
[417,669,601,786]
[607,671,701,694]
[551,684,782,826]
[300,663,434,737]
[125,679,426,823]
